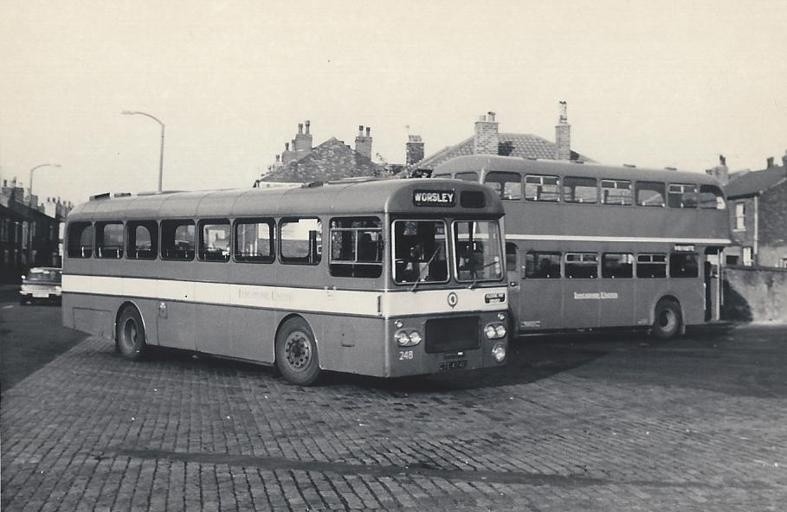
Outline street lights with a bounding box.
[122,108,165,193]
[30,163,61,195]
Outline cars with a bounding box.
[19,266,61,307]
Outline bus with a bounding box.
[427,154,738,341]
[60,170,513,387]
